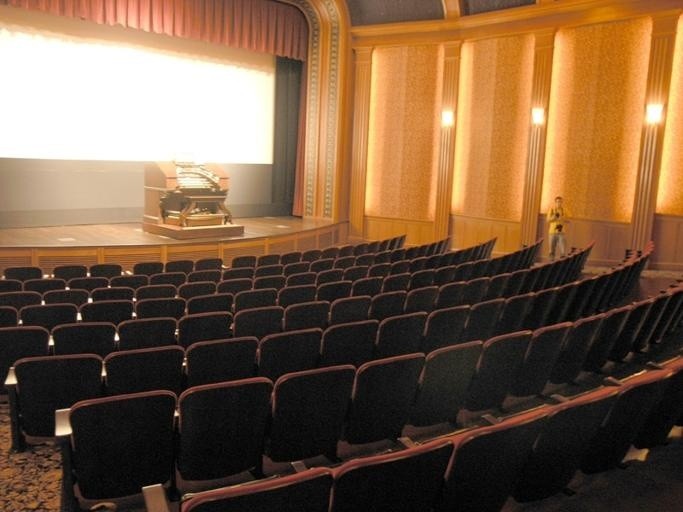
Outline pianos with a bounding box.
[144,161,230,226]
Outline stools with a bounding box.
[178,194,232,226]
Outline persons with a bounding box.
[547,196,569,259]
